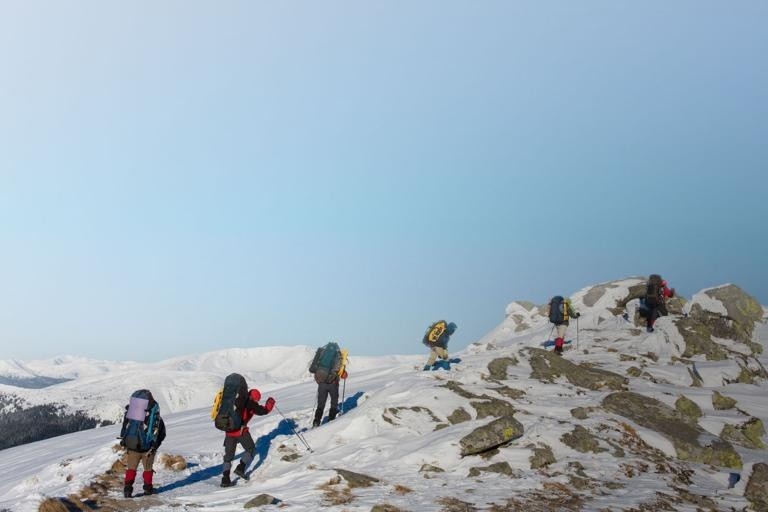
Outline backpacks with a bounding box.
[214,371,248,432]
[308,342,343,384]
[121,388,158,453]
[646,275,664,307]
[548,296,570,325]
[422,319,449,347]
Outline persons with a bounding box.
[219,389,276,487]
[553,297,580,356]
[646,277,675,332]
[119,397,166,498]
[309,343,348,427]
[423,322,457,371]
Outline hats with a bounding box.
[249,389,261,401]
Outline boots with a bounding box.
[221,470,234,487]
[234,459,249,480]
[123,485,133,497]
[143,483,156,494]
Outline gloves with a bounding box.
[341,370,348,379]
[260,397,276,414]
